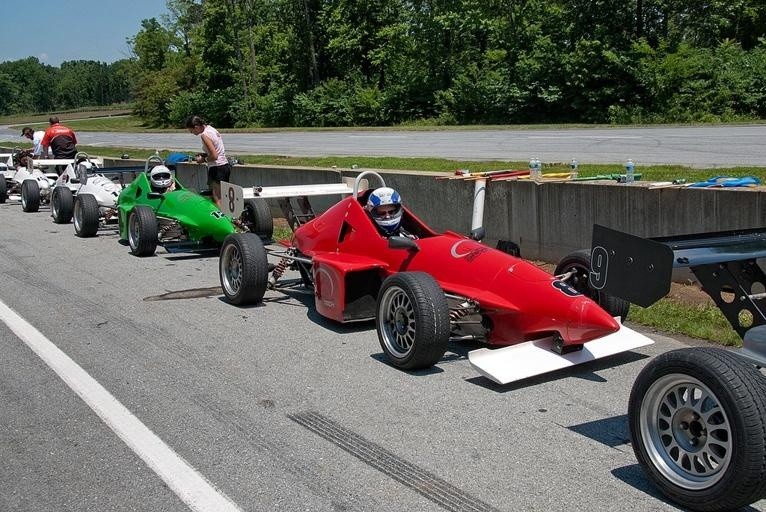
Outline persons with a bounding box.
[367,185,420,240]
[186,116,232,207]
[17,152,28,167]
[77,161,96,179]
[152,164,175,195]
[20,128,54,158]
[41,115,78,159]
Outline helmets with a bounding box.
[366,187,403,231]
[77,160,93,176]
[150,165,172,188]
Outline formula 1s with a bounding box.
[218,169,655,386]
[0,145,272,256]
[585,222,765,511]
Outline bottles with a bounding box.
[569,158,578,180]
[625,158,635,185]
[528,158,542,181]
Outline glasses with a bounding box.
[152,173,170,180]
[377,208,399,216]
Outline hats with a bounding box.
[21,127,31,135]
